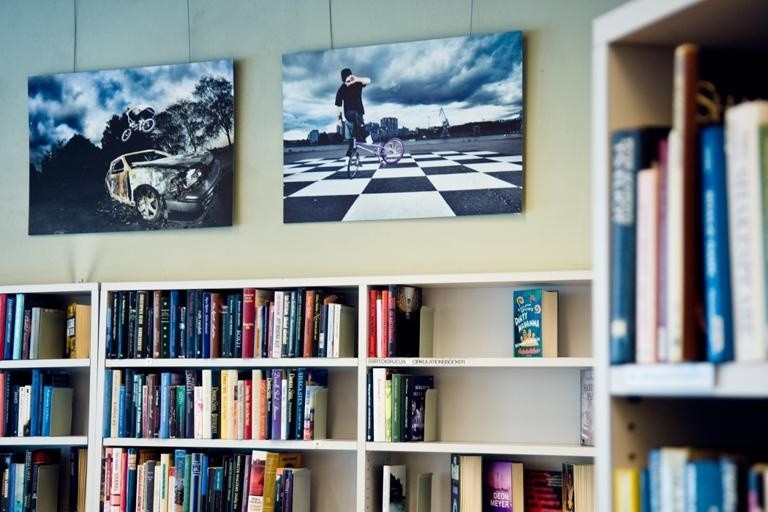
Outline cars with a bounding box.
[104,148,223,224]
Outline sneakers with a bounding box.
[346,151,359,156]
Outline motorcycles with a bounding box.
[121,105,157,143]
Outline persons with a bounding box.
[335,69,373,145]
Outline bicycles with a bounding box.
[338,112,405,179]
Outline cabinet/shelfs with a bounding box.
[1,282,100,512]
[590,0,768,512]
[360,269,595,512]
[93,274,362,512]
